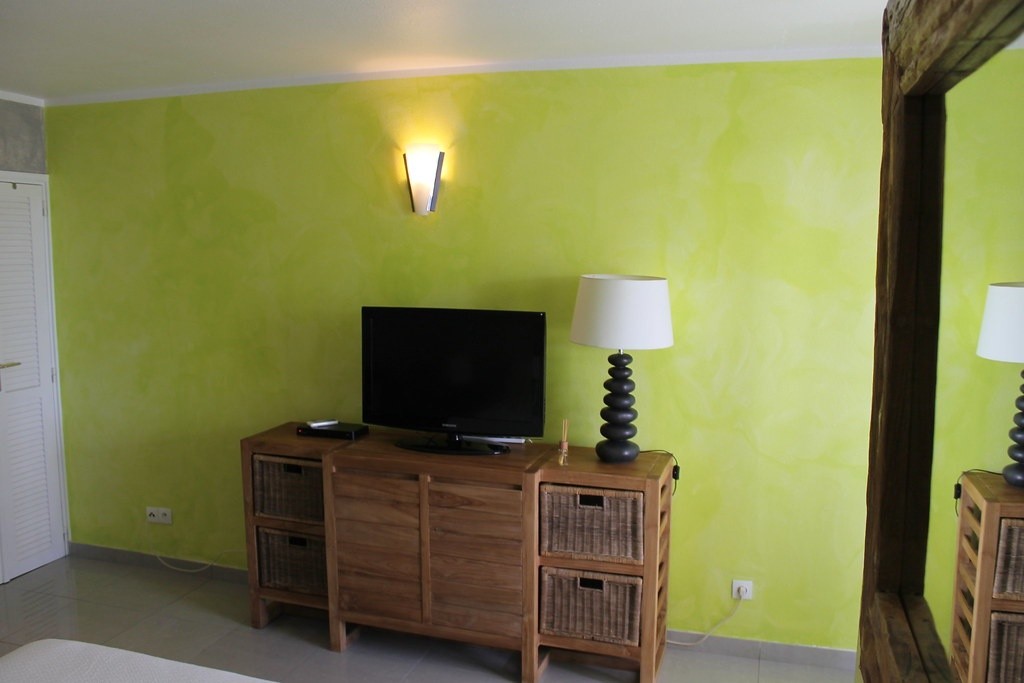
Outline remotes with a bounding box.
[307,420,337,427]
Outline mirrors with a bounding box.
[860,0,1024,683]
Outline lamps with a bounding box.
[403,148,444,213]
[975,284,1024,487]
[570,271,675,458]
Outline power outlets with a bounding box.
[731,580,752,601]
[146,507,158,523]
[159,508,172,524]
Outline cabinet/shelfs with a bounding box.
[239,420,676,683]
[954,471,1024,683]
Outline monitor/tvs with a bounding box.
[361,306,547,454]
